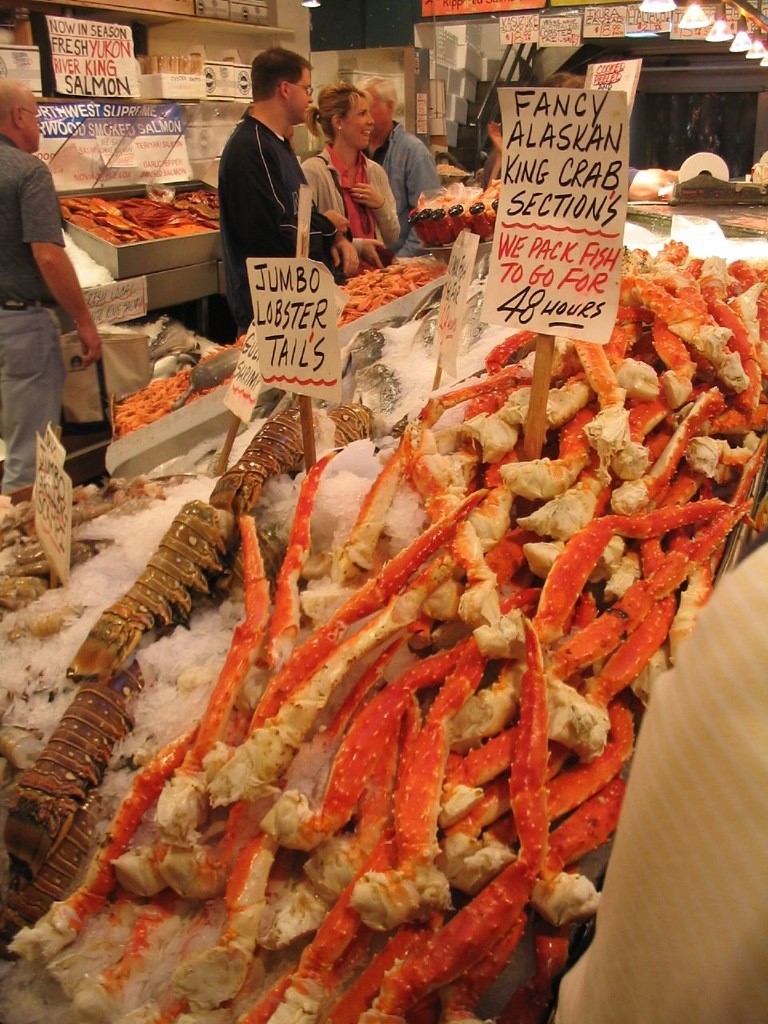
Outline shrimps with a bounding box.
[68,500,240,678]
[0,652,145,952]
[326,401,379,451]
[207,394,321,520]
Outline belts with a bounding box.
[0,298,59,309]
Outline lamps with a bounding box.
[302,0,321,7]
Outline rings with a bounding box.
[360,194,363,198]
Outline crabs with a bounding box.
[8,238,768,1024]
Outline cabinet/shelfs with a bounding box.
[33,0,295,104]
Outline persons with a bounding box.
[357,76,441,258]
[482,73,674,202]
[0,79,102,509]
[300,82,400,276]
[218,48,357,341]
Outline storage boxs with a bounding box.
[230,0,269,26]
[203,66,256,104]
[141,73,207,101]
[61,178,230,312]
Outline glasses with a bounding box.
[278,79,313,96]
[11,107,41,124]
